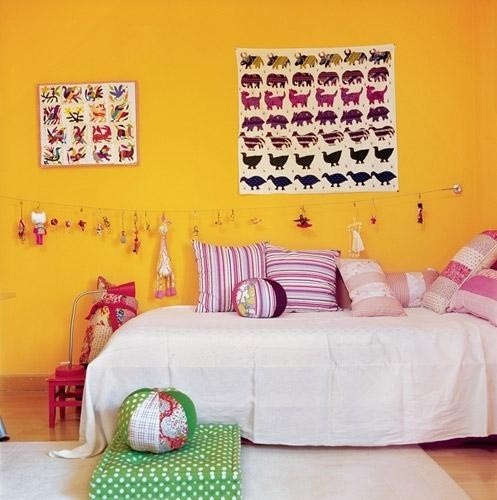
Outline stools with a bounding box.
[46,374,86,427]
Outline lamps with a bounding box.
[55,281,135,378]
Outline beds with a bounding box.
[88,306,497,448]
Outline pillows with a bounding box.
[446,267,497,327]
[265,244,341,312]
[419,231,497,314]
[232,278,287,318]
[333,255,408,317]
[117,386,197,454]
[336,270,439,308]
[192,240,267,312]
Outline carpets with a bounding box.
[0,440,475,500]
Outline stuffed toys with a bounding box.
[154,218,177,300]
